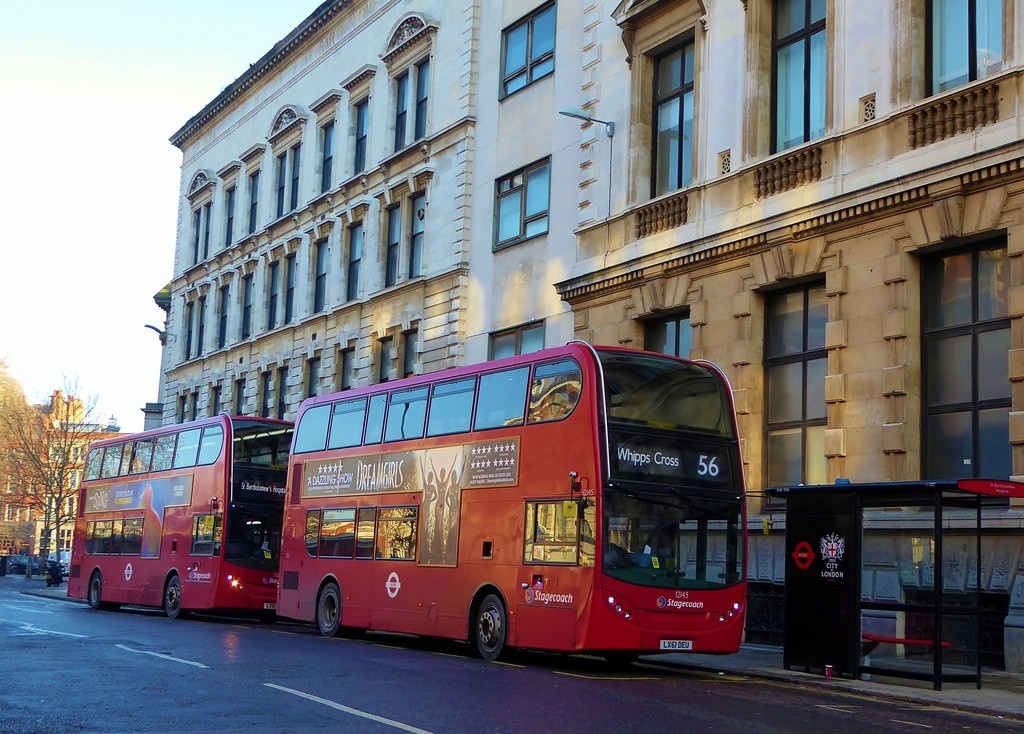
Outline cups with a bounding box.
[825,665,833,680]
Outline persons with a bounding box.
[44,563,63,588]
[646,500,687,566]
[217,500,224,512]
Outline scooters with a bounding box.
[44,559,64,587]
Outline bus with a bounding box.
[67,411,296,620]
[276,340,749,661]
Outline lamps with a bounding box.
[558,103,614,137]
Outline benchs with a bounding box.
[863,632,953,658]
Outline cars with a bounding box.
[6,555,39,575]
